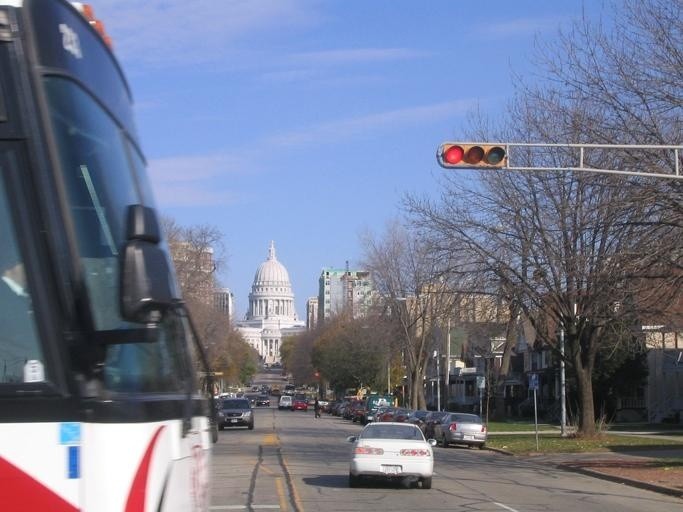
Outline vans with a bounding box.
[362,396,392,424]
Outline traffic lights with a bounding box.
[443,143,504,168]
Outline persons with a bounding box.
[314,397,321,418]
[0,261,40,351]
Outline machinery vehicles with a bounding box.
[272,384,281,395]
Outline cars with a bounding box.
[374,407,487,448]
[278,393,315,411]
[318,396,366,422]
[214,384,271,431]
[346,422,437,489]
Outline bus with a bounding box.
[0,2,217,511]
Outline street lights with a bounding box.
[395,297,440,412]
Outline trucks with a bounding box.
[283,384,296,395]
